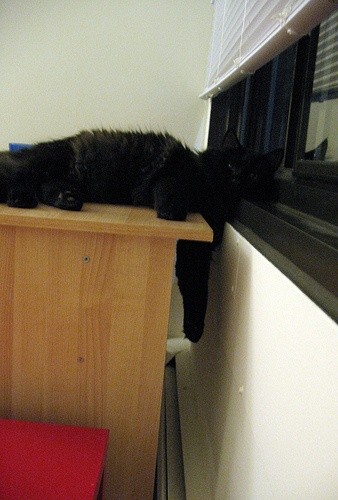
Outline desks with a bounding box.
[0,199,214,499]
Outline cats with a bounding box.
[303,137,328,161]
[0,127,284,354]
